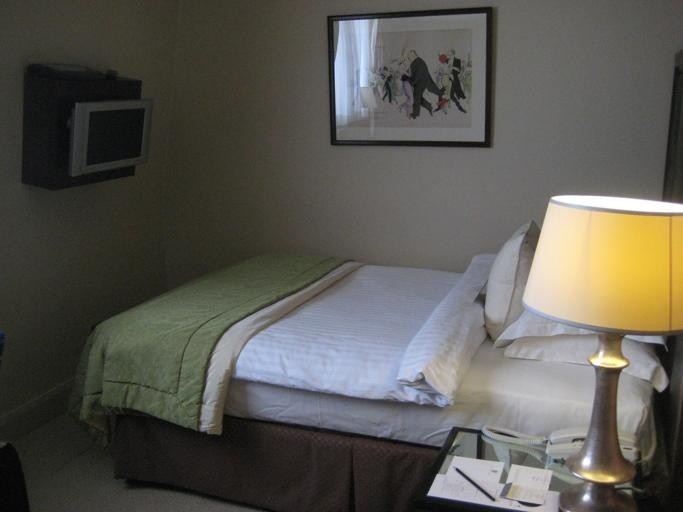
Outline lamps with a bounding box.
[521,194,683,486]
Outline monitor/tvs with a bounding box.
[66,98,154,175]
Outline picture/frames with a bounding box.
[327,7,495,149]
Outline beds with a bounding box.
[70,250,683,512]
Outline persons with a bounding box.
[370,47,471,120]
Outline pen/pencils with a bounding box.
[455,467,495,501]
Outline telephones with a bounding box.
[545,426,640,463]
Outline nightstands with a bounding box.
[409,425,683,512]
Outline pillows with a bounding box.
[486,220,671,393]
